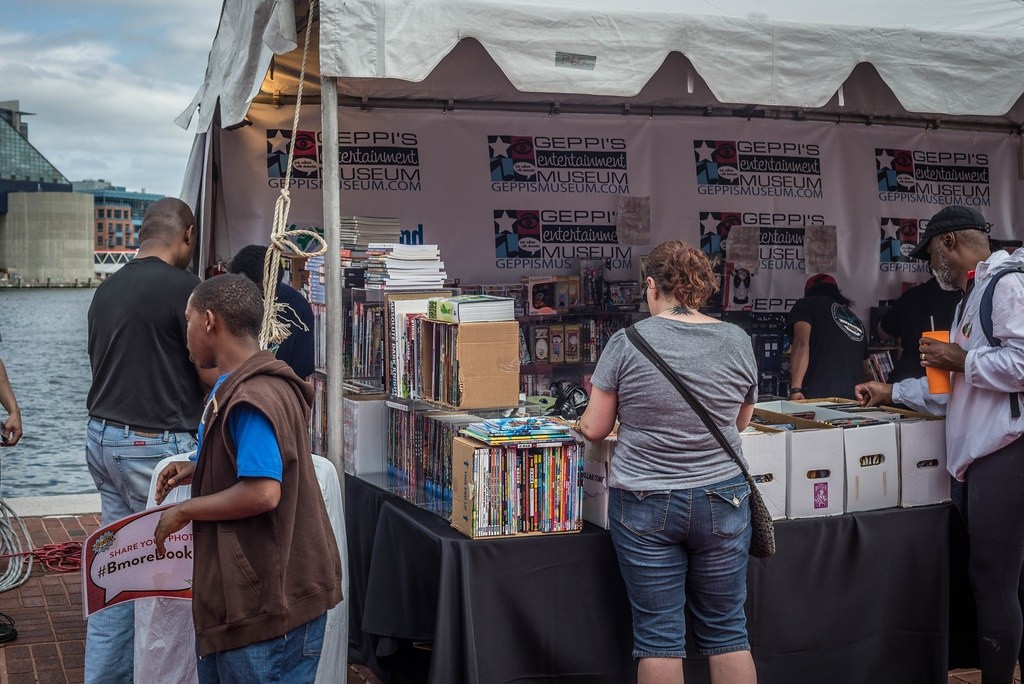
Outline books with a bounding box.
[739,401,927,483]
[864,350,894,385]
[580,317,630,363]
[299,214,583,537]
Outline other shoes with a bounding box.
[0,624,18,643]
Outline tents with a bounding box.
[172,0,1024,509]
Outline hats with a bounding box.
[805,274,837,290]
[909,205,992,259]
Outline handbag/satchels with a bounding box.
[747,483,777,558]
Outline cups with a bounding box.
[923,331,951,394]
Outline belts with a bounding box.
[89,416,192,434]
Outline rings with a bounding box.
[921,353,925,360]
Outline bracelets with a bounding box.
[789,387,802,395]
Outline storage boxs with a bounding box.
[281,253,308,290]
[341,392,389,476]
[418,318,952,541]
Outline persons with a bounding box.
[154,274,340,684]
[84,198,221,684]
[220,244,315,396]
[855,205,1024,684]
[0,332,23,644]
[579,240,759,684]
[786,273,871,403]
